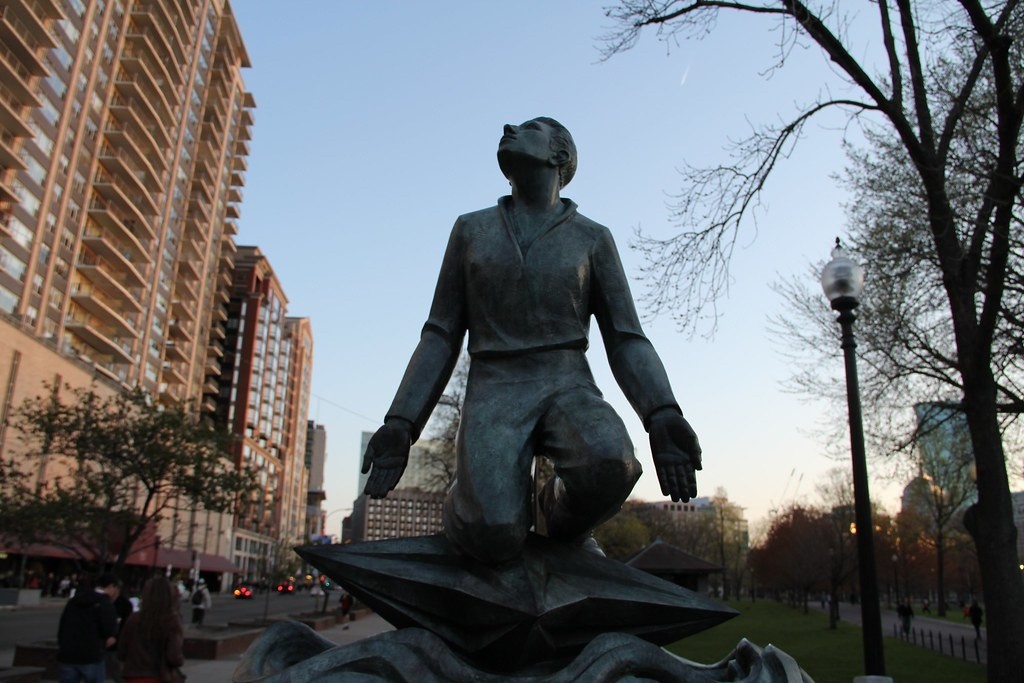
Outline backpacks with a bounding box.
[191,587,206,605]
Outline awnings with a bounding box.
[156,547,239,571]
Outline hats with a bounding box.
[198,578,205,583]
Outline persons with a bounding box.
[897,595,914,634]
[967,599,983,640]
[190,578,211,624]
[24,571,79,599]
[339,591,353,630]
[361,115,704,564]
[56,569,186,683]
[820,591,832,608]
[922,603,932,615]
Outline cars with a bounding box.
[234,584,256,599]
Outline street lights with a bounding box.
[822,237,891,682]
